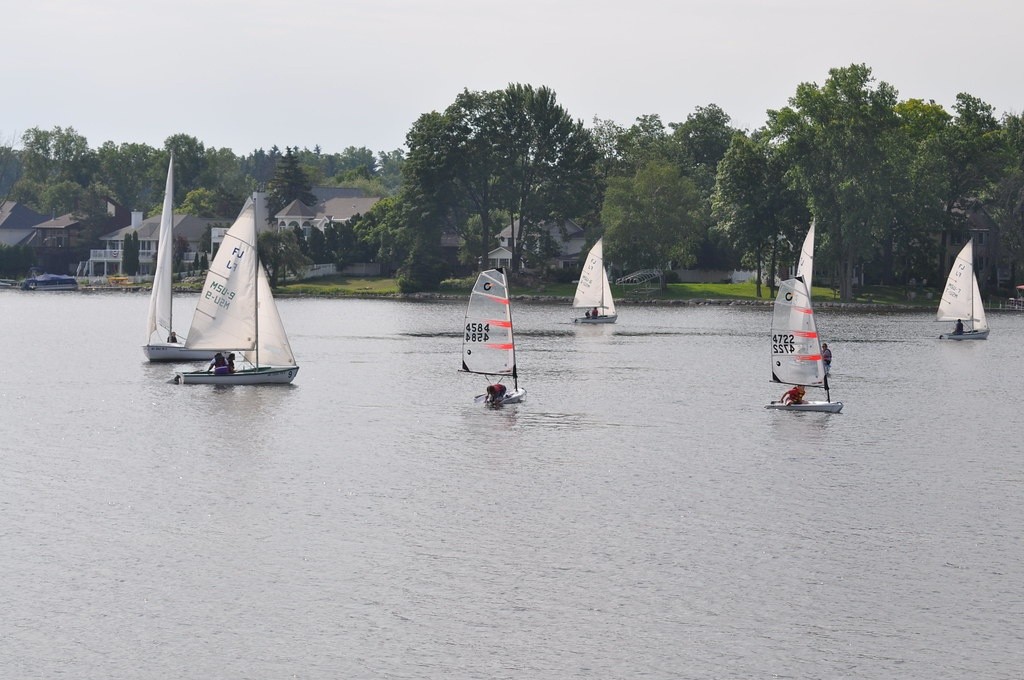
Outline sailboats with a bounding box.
[572,236,619,326]
[782,216,831,377]
[458,265,529,404]
[170,196,300,385]
[933,236,990,340]
[21,273,79,291]
[143,152,232,362]
[769,275,843,413]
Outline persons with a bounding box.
[167,331,177,343]
[779,385,808,406]
[227,353,236,373]
[485,384,507,405]
[953,319,963,335]
[207,352,230,374]
[591,307,598,319]
[821,343,832,365]
